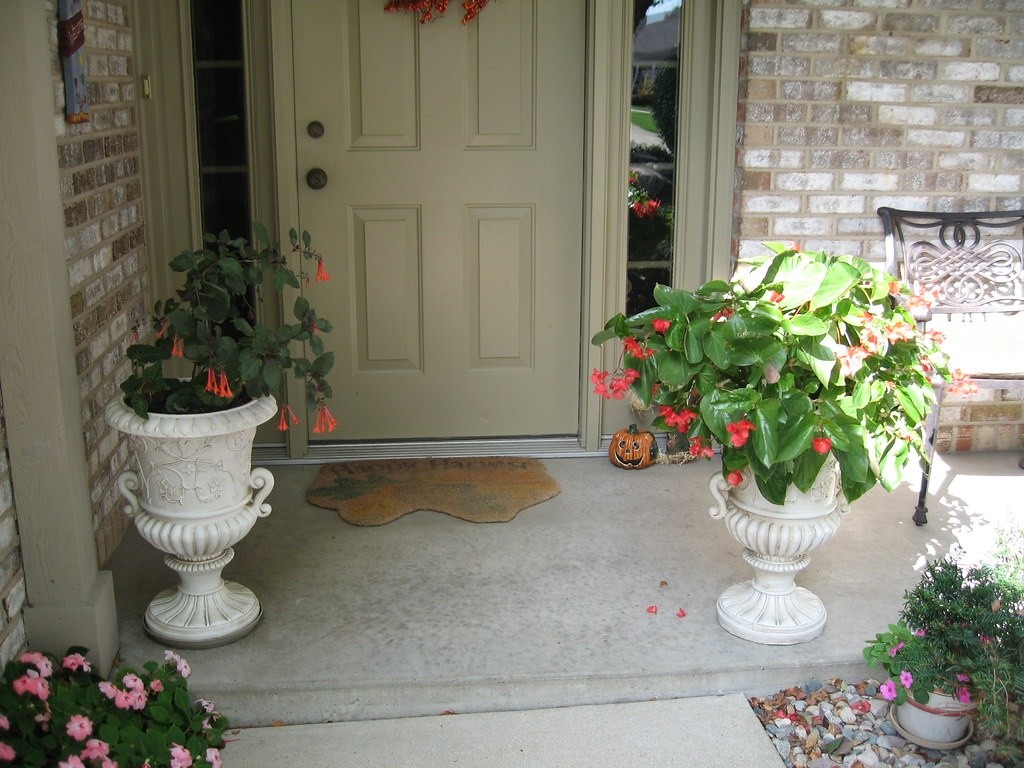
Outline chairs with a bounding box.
[878,207,1023,527]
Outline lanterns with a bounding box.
[607,424,658,470]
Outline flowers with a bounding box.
[860,552,1023,742]
[588,238,980,505]
[1,641,230,767]
[121,225,338,434]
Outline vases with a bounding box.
[708,446,851,645]
[105,378,277,649]
[890,676,974,748]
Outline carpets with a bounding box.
[305,456,558,526]
